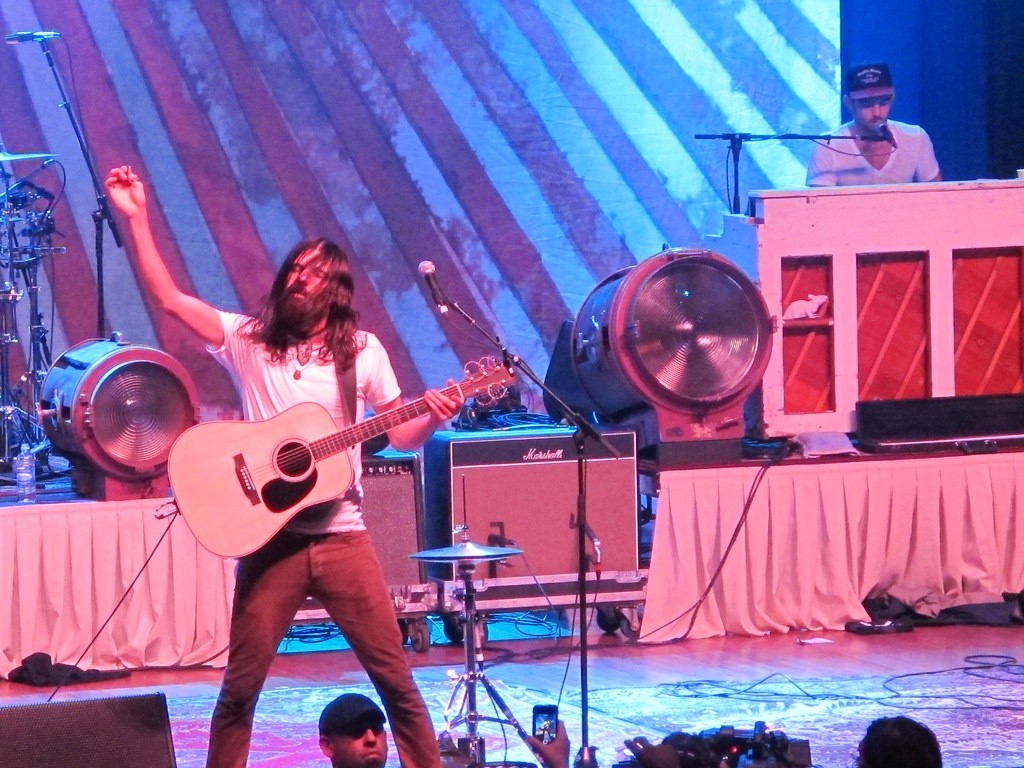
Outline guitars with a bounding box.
[165,356,516,558]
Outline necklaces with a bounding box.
[288,336,324,380]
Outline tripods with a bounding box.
[444,473,550,768]
[0,144,53,464]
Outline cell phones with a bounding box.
[532,704,558,745]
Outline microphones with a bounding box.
[5,32,61,45]
[418,260,448,315]
[0,160,53,202]
[488,533,515,545]
[874,121,898,148]
[586,522,601,546]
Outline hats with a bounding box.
[846,63,894,98]
[319,693,386,731]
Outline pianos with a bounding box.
[702,169,1024,439]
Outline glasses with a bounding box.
[344,720,383,736]
[858,95,892,108]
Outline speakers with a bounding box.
[360,453,423,587]
[0,691,177,768]
[423,423,638,584]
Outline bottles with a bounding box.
[15,443,36,494]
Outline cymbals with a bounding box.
[0,152,60,161]
[407,541,524,565]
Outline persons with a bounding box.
[319,692,389,768]
[104,164,466,768]
[805,61,941,188]
[855,715,945,768]
